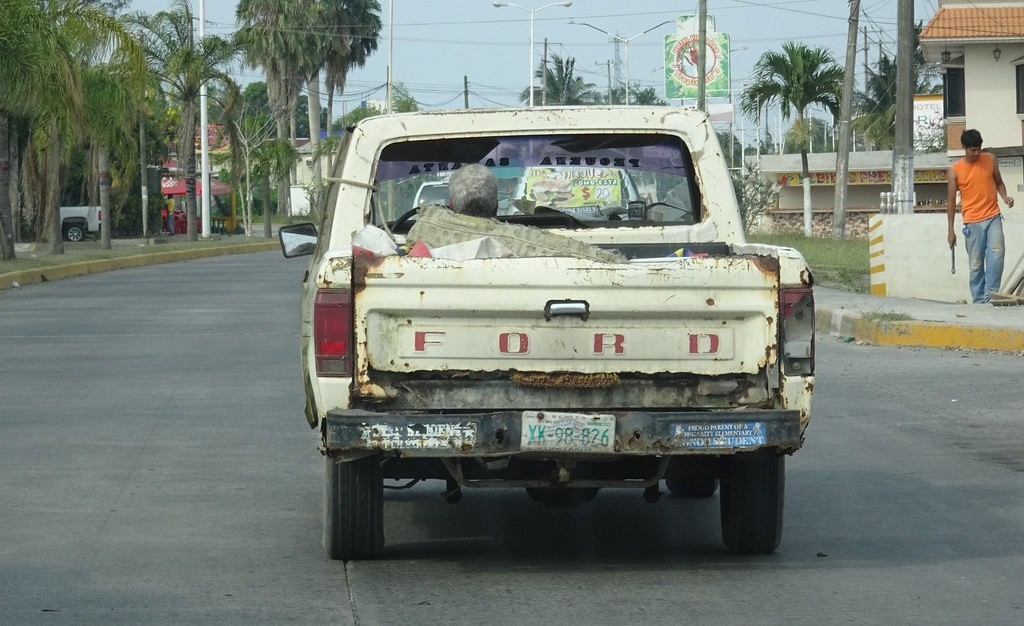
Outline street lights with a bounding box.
[569,21,678,105]
[493,1,572,107]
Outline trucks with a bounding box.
[276,105,818,560]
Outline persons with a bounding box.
[448,164,499,220]
[165,194,175,235]
[947,129,1014,304]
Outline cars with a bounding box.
[510,166,639,222]
[413,179,450,209]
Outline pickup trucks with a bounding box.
[61,205,102,241]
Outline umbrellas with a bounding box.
[160,179,230,216]
